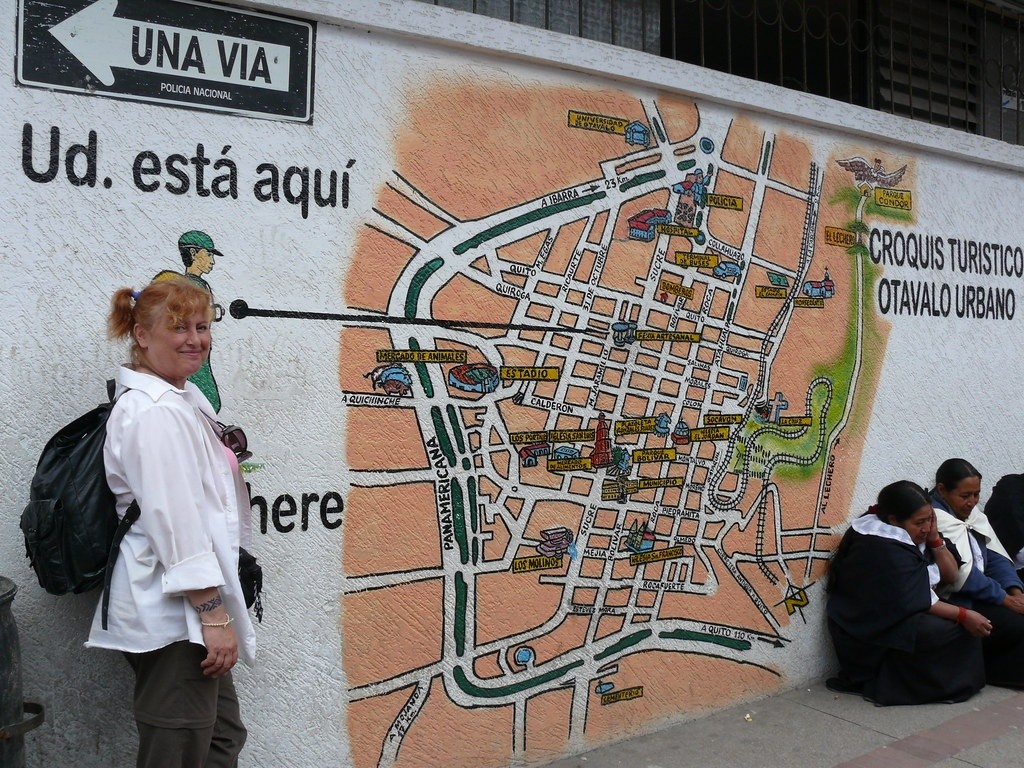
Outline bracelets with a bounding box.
[957,606,966,622]
[928,537,942,547]
[201,613,234,628]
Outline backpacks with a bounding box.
[19,378,141,632]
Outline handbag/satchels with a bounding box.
[238,545,264,625]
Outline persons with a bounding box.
[83,278,258,768]
[823,458,1024,707]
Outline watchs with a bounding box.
[928,539,946,551]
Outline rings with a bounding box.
[1013,601,1016,602]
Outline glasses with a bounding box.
[198,407,253,464]
